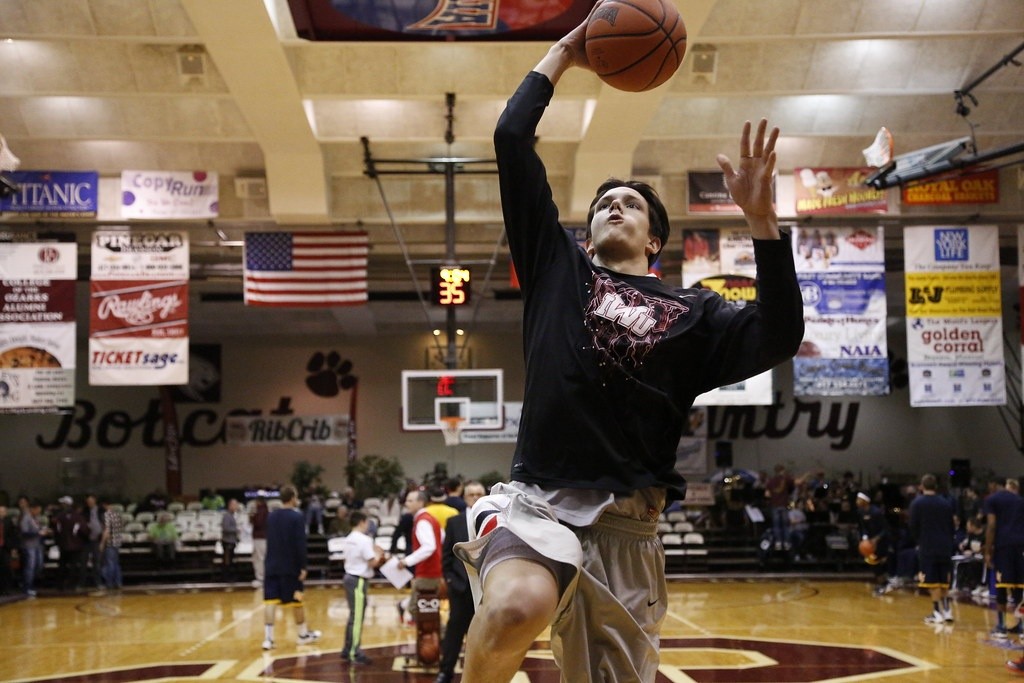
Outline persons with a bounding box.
[249,495,269,587]
[203,487,225,509]
[460,0,805,683]
[148,513,178,571]
[144,486,168,510]
[220,498,242,592]
[709,463,1024,675]
[263,485,322,649]
[0,493,123,600]
[303,473,486,683]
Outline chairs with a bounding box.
[8,494,406,568]
[658,511,708,555]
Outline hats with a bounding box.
[58,495,74,505]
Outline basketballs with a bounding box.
[584,0,688,94]
[858,541,875,556]
[419,639,439,664]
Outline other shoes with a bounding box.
[942,608,954,621]
[1008,627,1024,634]
[1005,656,1024,675]
[990,625,1008,638]
[877,585,894,595]
[924,614,943,623]
[435,672,454,683]
[340,650,373,665]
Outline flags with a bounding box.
[242,230,372,303]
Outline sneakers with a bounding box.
[262,640,275,649]
[297,630,321,644]
